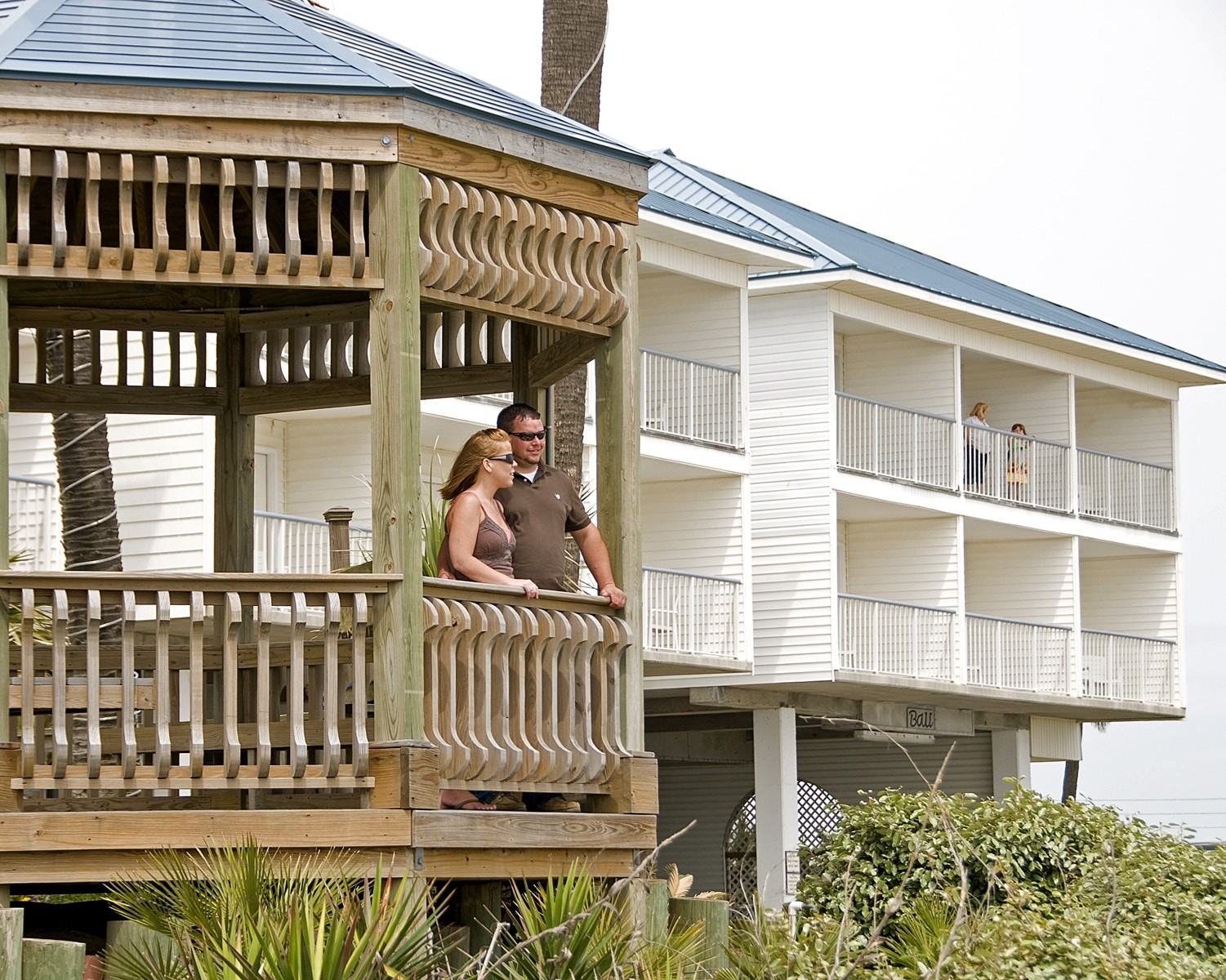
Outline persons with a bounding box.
[437,403,628,812]
[1007,423,1036,501]
[963,403,992,494]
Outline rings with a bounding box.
[530,587,534,591]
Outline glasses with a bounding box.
[487,454,514,464]
[509,429,547,441]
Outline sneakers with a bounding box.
[541,797,581,812]
[488,793,527,811]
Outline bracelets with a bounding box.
[438,571,446,578]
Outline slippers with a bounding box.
[439,790,497,811]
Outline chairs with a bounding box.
[649,608,680,655]
[1082,655,1120,696]
[841,636,856,672]
[968,666,984,687]
[1076,483,1108,517]
[646,403,666,431]
